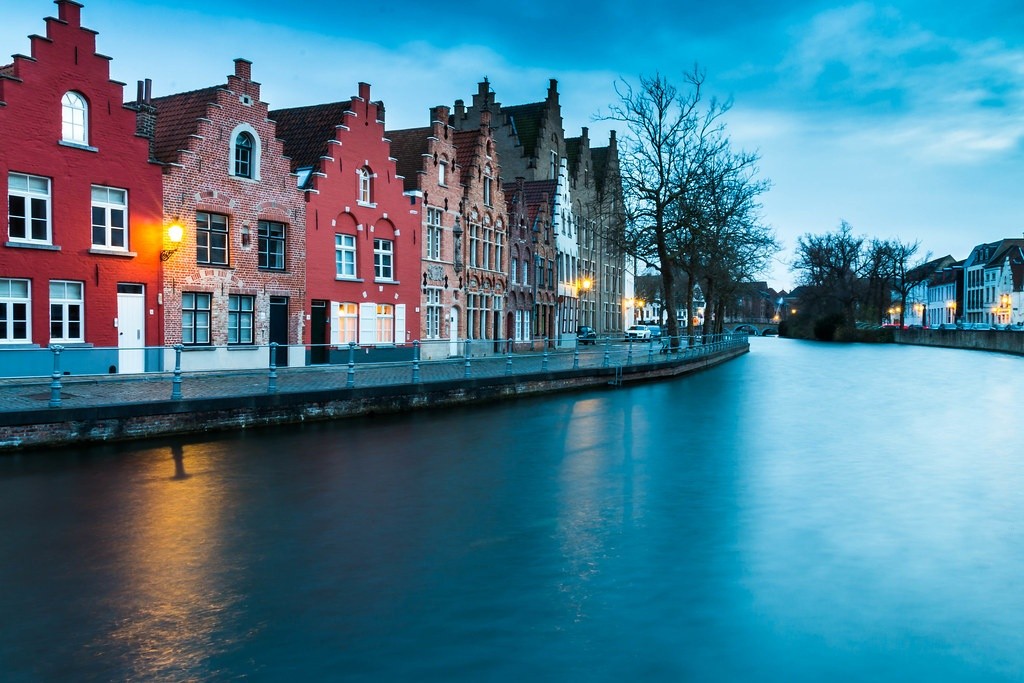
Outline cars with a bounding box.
[578,326,597,345]
[624,325,652,342]
[648,325,662,341]
[855,320,1024,331]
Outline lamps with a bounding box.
[161,226,183,261]
